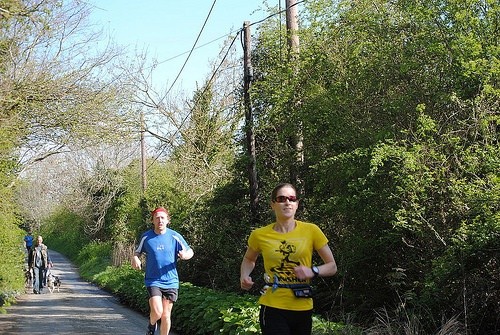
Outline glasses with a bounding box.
[273,195,297,203]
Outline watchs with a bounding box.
[312,265,319,277]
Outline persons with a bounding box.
[27,235,54,295]
[239,182,338,335]
[24,233,34,253]
[130,207,194,335]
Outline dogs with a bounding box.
[46,272,62,294]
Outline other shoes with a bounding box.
[33,289,39,294]
[146,323,156,335]
